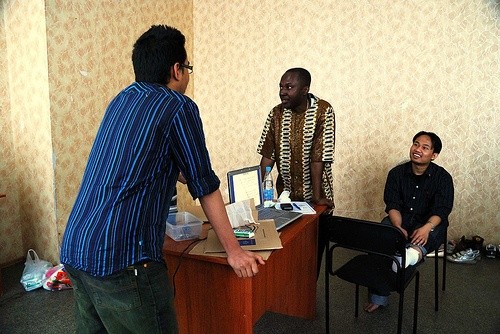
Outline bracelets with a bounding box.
[427,221,434,232]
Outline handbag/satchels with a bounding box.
[21,249,53,292]
[453,235,484,255]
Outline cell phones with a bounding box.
[280,203,293,211]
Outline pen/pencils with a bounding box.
[293,203,301,210]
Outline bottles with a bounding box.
[263,166,273,207]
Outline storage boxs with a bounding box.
[165,211,204,242]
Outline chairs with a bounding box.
[378,214,449,314]
[318,212,420,334]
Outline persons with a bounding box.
[60,25,265,334]
[256,68,335,282]
[362,129,454,314]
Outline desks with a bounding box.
[163,204,327,334]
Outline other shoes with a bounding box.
[426,240,456,258]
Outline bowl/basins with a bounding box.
[165,211,204,241]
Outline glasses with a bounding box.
[181,63,193,74]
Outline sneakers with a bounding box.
[447,248,480,264]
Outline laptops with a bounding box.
[227,165,303,230]
